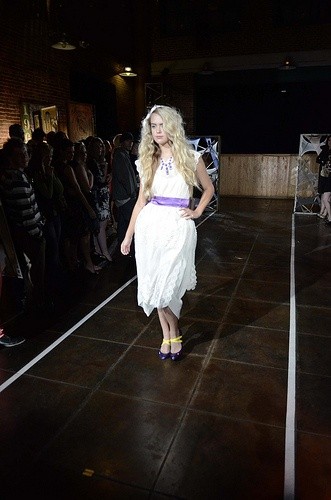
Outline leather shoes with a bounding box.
[0,333,25,347]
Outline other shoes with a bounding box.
[317,214,326,218]
[84,264,104,275]
[324,220,331,224]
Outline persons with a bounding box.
[0,123,138,346]
[317,135,331,224]
[121,104,214,361]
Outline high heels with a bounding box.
[159,328,183,362]
[93,252,112,264]
